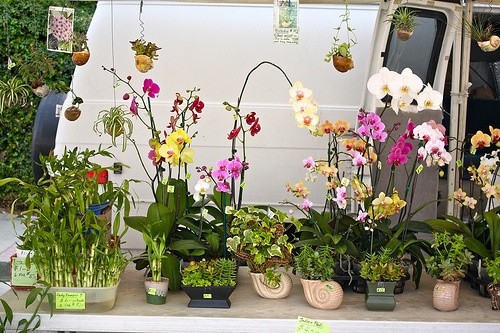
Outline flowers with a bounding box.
[102,66,500,298]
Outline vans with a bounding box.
[27,2,499,233]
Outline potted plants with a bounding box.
[324,0,358,72]
[384,6,419,41]
[123,203,176,305]
[294,243,344,311]
[130,38,161,72]
[179,258,237,309]
[440,4,500,52]
[92,105,134,151]
[423,228,475,312]
[484,250,500,310]
[71,31,90,65]
[226,205,303,299]
[359,247,406,312]
